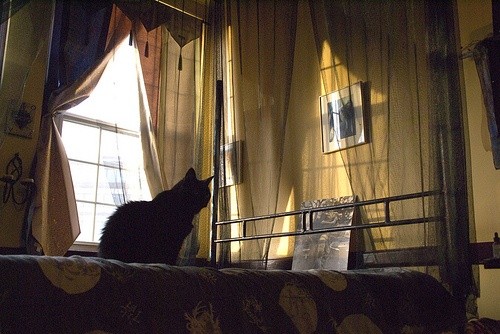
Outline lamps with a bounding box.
[1,151,35,206]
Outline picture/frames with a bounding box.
[211,139,242,189]
[319,81,368,155]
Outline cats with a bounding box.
[96,167,216,265]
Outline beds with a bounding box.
[1,50,465,334]
